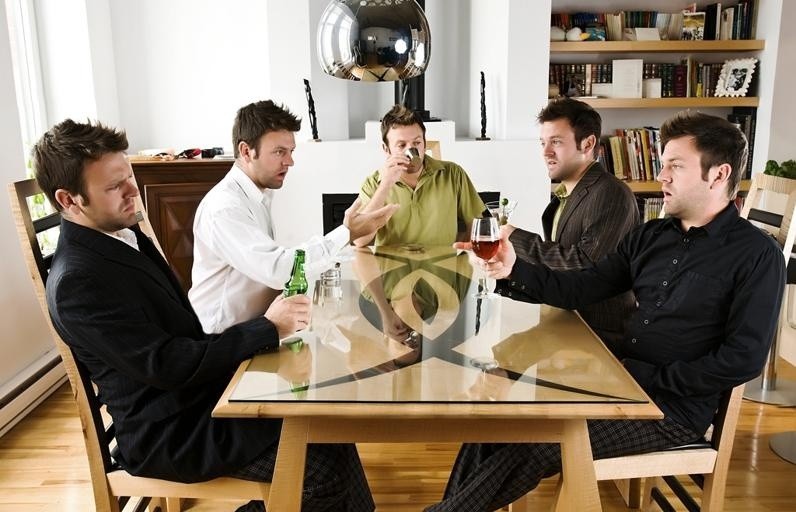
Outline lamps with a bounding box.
[314,0,432,83]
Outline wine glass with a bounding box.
[469,357,499,401]
[471,218,500,301]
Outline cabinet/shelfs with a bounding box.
[548,38,765,223]
[125,156,236,295]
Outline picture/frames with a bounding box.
[715,56,760,98]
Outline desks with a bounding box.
[209,235,664,512]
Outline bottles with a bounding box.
[284,249,308,332]
[281,337,309,400]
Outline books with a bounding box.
[597,126,667,222]
[549,56,723,98]
[552,1,754,41]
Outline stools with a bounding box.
[736,258,795,411]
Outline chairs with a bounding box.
[320,188,502,240]
[6,168,290,511]
[542,170,795,512]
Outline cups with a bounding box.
[320,262,342,285]
[321,286,342,305]
[485,200,519,225]
[403,147,422,174]
[404,331,419,345]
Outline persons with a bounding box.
[344,103,496,248]
[29,115,378,512]
[735,67,748,92]
[353,247,474,369]
[497,99,640,270]
[419,109,785,512]
[728,68,738,92]
[186,99,402,334]
[244,338,314,385]
[446,312,598,402]
